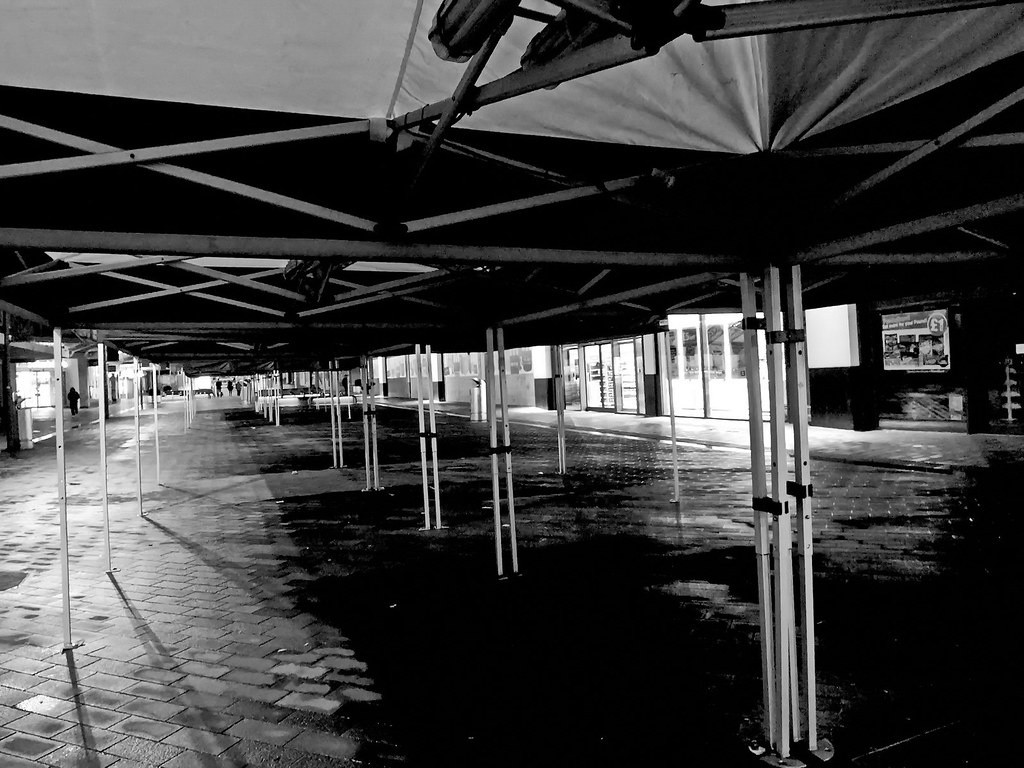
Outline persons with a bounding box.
[68,387,80,415]
[342,374,347,394]
[235,382,242,396]
[228,381,233,395]
[211,377,222,397]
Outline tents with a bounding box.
[0,0,1024,768]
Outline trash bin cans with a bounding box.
[18,408,34,450]
[468,377,487,423]
